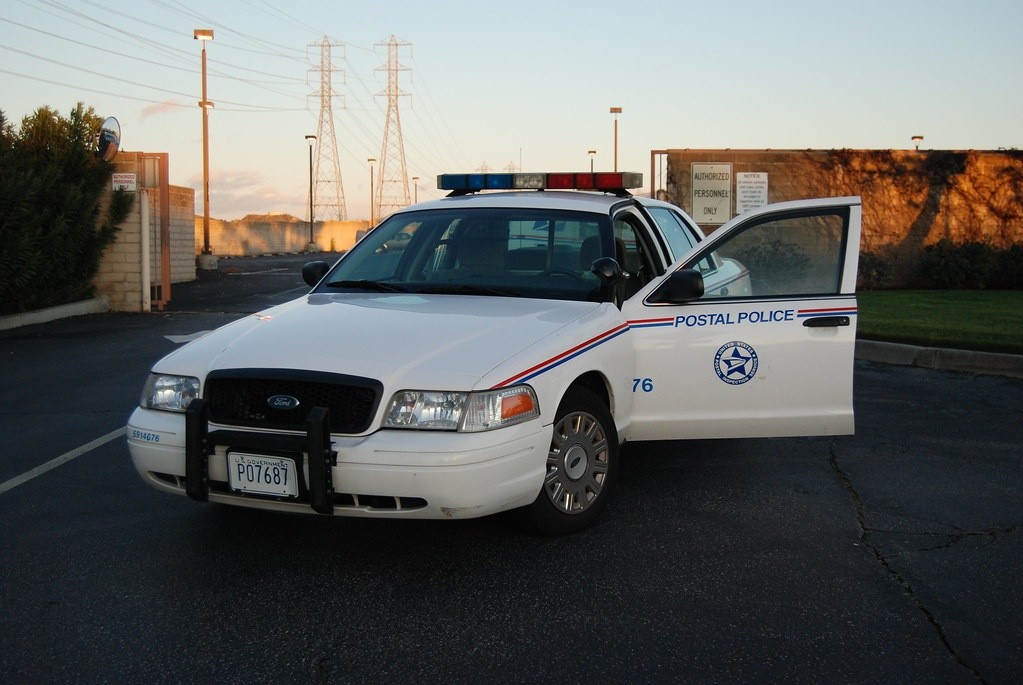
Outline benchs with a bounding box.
[510,244,642,274]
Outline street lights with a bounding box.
[588,150,597,173]
[368,159,375,229]
[412,177,419,205]
[192,28,218,271]
[608,106,623,173]
[305,135,318,251]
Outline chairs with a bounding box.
[435,222,510,282]
[579,234,633,300]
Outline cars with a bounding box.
[125,172,863,542]
